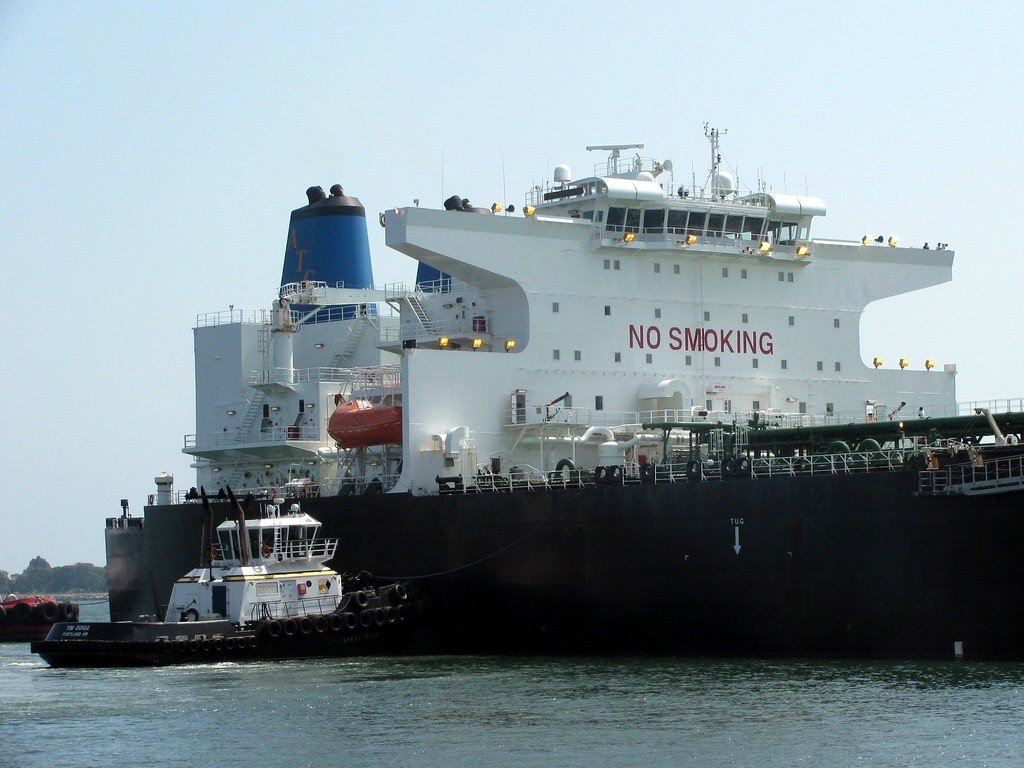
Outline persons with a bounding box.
[2,590,43,604]
[918,407,925,420]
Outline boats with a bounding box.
[0,595,82,645]
[30,495,399,672]
[325,400,403,448]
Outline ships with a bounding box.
[105,121,1024,674]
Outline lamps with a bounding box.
[872,357,883,368]
[523,205,536,218]
[438,336,449,350]
[758,242,770,252]
[899,359,909,370]
[504,339,516,353]
[925,360,934,371]
[862,235,874,246]
[888,236,898,247]
[491,203,503,215]
[471,338,482,352]
[624,232,635,242]
[796,246,807,255]
[685,234,697,244]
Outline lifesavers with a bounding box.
[270,488,277,498]
[186,608,199,622]
